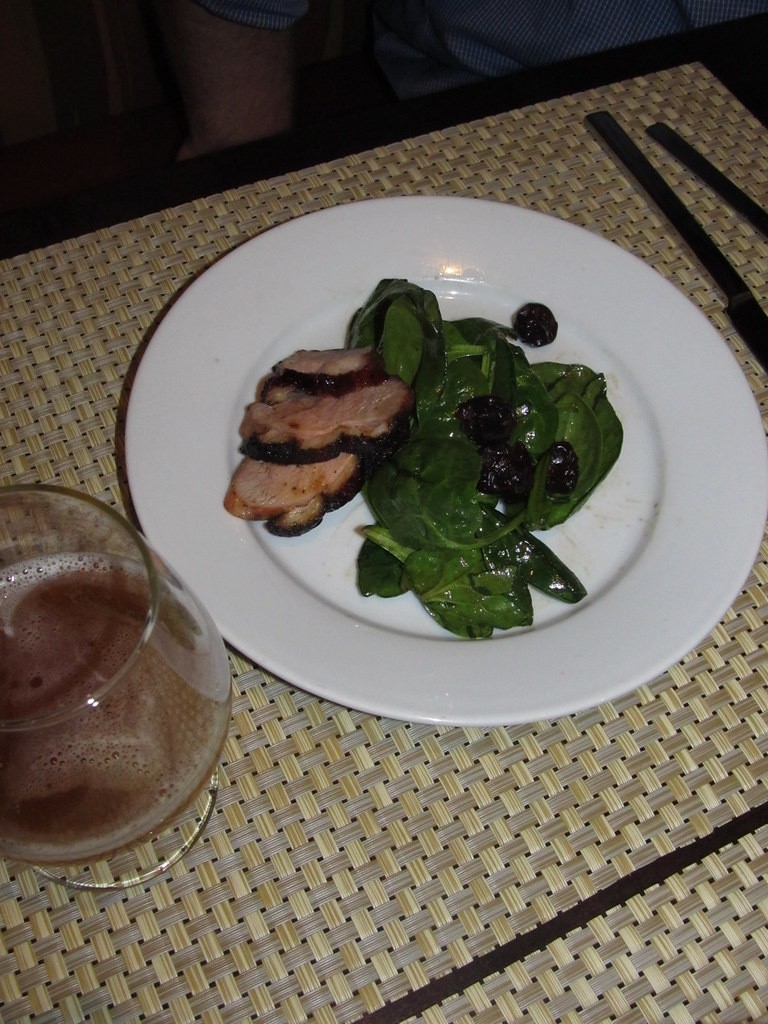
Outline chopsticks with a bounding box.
[582,106,768,381]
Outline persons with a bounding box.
[169,0,768,163]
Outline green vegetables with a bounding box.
[343,278,624,640]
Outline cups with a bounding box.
[0,484,231,889]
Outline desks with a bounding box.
[0,5,768,1024]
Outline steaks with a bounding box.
[224,345,418,537]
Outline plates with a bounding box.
[125,195,768,727]
